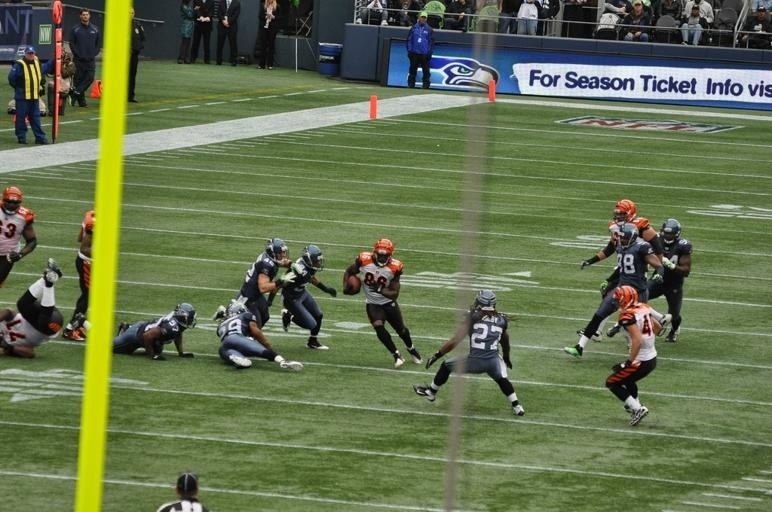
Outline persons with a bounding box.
[67,303,197,361]
[256,0,280,70]
[127,7,147,103]
[0,258,63,358]
[597,0,715,44]
[564,199,693,428]
[739,7,772,49]
[355,1,588,37]
[0,186,37,288]
[156,474,208,512]
[406,12,434,88]
[413,288,526,415]
[63,211,95,342]
[174,0,241,66]
[8,46,50,145]
[343,238,425,369]
[68,8,102,107]
[210,238,336,372]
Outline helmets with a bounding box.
[618,222,639,249]
[613,285,639,309]
[301,245,325,272]
[658,218,682,244]
[175,303,197,329]
[373,239,394,266]
[474,288,497,311]
[266,237,288,264]
[2,186,23,211]
[226,301,248,318]
[614,198,637,225]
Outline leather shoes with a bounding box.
[17,138,28,144]
[34,136,49,144]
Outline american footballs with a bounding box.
[348,275,361,292]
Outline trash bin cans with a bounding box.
[318,42,344,75]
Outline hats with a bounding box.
[24,45,34,57]
[420,10,427,18]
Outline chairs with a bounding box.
[361,0,772,48]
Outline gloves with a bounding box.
[612,359,632,373]
[600,280,610,297]
[581,259,593,268]
[179,352,194,357]
[424,356,438,370]
[152,353,164,360]
[504,358,512,369]
[326,279,383,297]
[279,272,297,285]
[652,256,677,285]
[7,250,23,262]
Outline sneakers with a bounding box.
[226,356,253,368]
[47,258,61,278]
[72,312,86,329]
[658,314,681,342]
[306,341,329,350]
[393,354,406,368]
[406,345,422,363]
[627,406,648,425]
[62,328,84,341]
[212,306,226,320]
[44,269,58,283]
[511,404,525,416]
[564,324,621,358]
[413,384,436,402]
[281,309,292,332]
[280,360,303,371]
